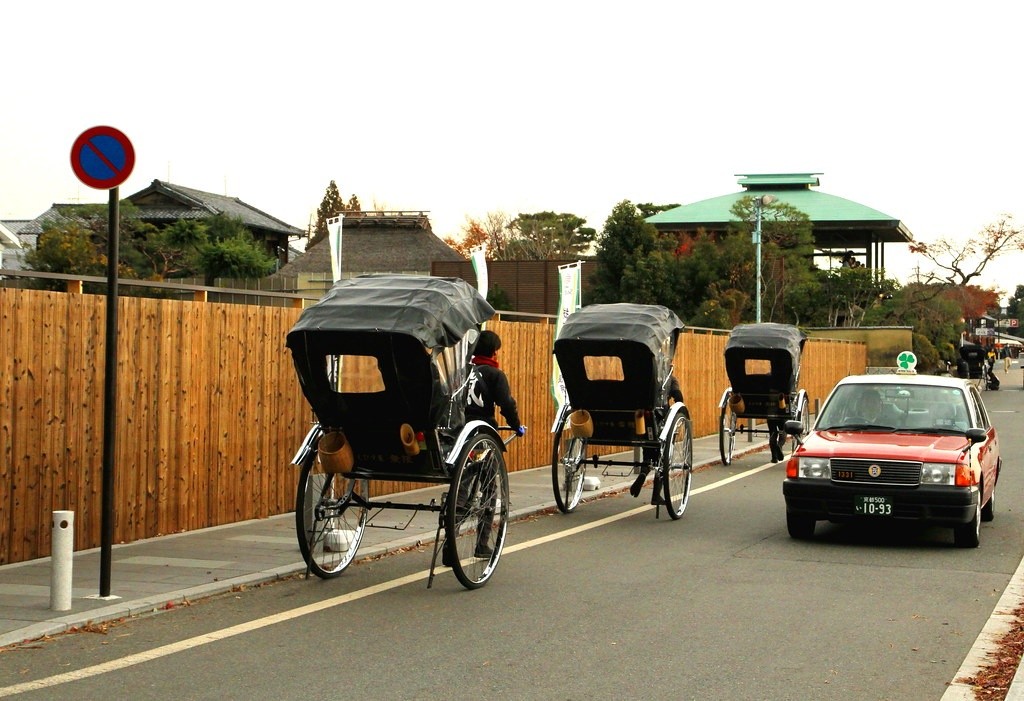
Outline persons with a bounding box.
[766,417,791,463]
[841,250,867,270]
[985,370,1000,390]
[986,347,996,372]
[1002,343,1012,374]
[631,375,685,506]
[440,329,526,569]
[846,388,888,426]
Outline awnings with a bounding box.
[994,338,1023,348]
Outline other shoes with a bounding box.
[628,474,644,497]
[443,550,452,567]
[650,485,668,506]
[770,444,785,464]
[475,547,494,559]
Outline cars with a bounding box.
[958,346,989,391]
[783,351,1002,547]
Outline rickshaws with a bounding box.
[550,304,694,522]
[286,271,526,591]
[718,322,811,467]
